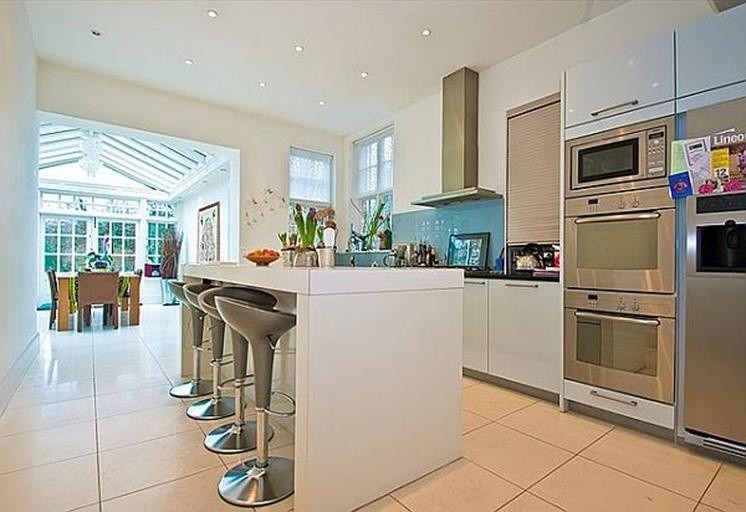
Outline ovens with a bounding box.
[564,187,677,407]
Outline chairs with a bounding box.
[39,267,142,334]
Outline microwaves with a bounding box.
[570,124,669,190]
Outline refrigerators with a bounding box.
[682,96,745,456]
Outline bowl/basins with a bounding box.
[245,256,280,266]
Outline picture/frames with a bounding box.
[196,201,221,264]
[446,231,491,270]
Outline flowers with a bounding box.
[276,202,338,249]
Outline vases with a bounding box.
[290,251,316,268]
[317,250,338,269]
[279,248,296,266]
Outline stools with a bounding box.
[181,281,249,423]
[211,294,296,509]
[195,285,277,456]
[164,277,211,400]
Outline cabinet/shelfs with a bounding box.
[489,278,561,397]
[563,1,746,130]
[461,281,486,377]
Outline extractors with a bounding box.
[411,66,503,207]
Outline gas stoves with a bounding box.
[462,267,491,276]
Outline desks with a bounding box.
[178,260,467,512]
